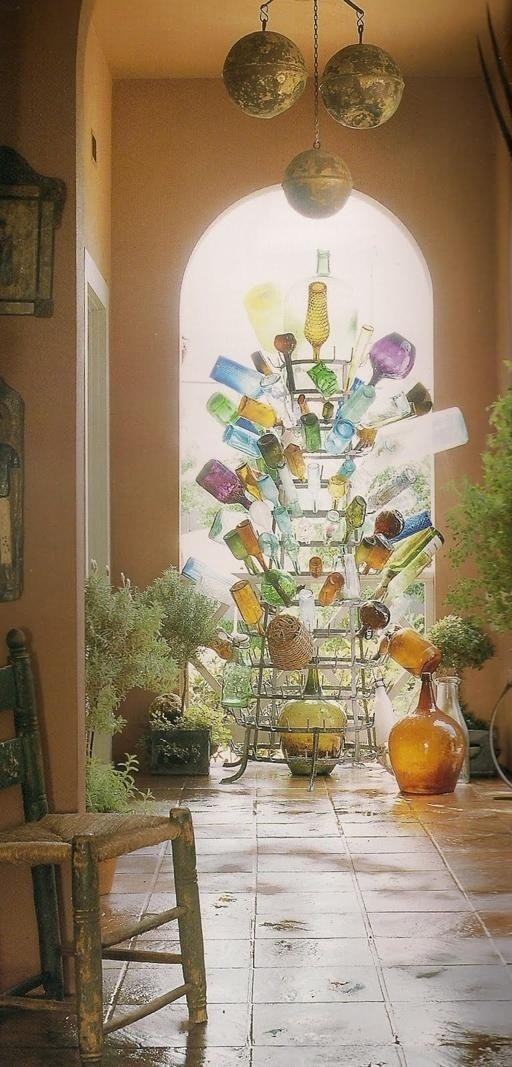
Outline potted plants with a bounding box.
[425,609,495,776]
[131,563,231,781]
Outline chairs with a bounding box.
[0,624,210,1067]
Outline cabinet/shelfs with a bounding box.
[219,340,400,787]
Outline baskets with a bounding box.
[267,616,313,671]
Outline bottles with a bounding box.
[276,658,348,776]
[434,676,471,785]
[219,631,254,709]
[194,319,470,642]
[316,248,331,277]
[384,625,444,680]
[303,281,330,364]
[387,672,467,795]
